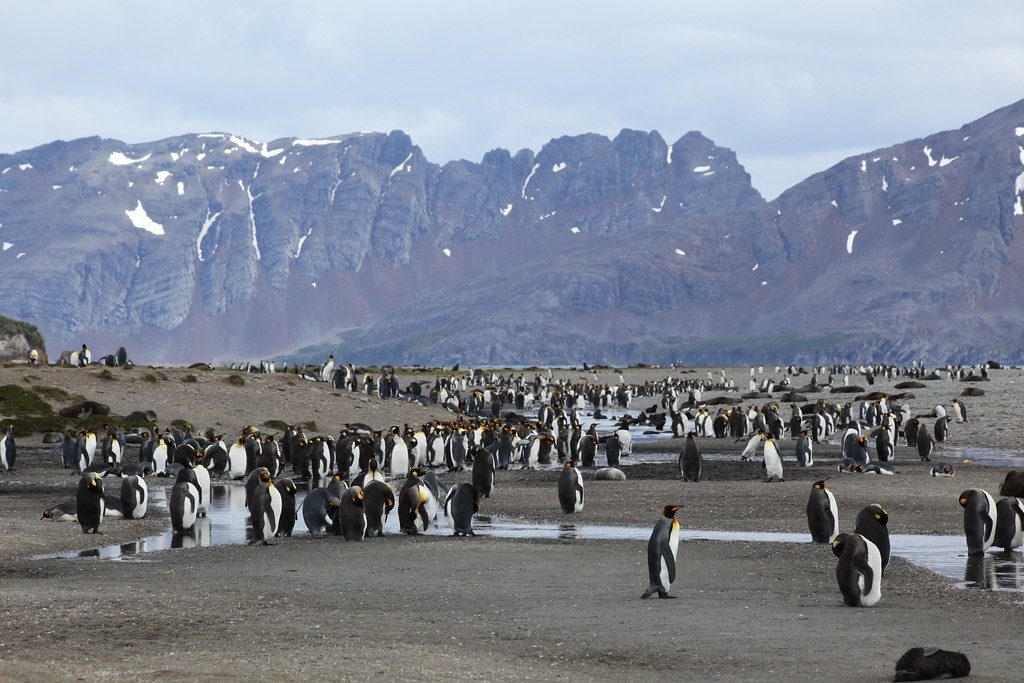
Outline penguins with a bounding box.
[889,647,972,682]
[0,343,1024,608]
